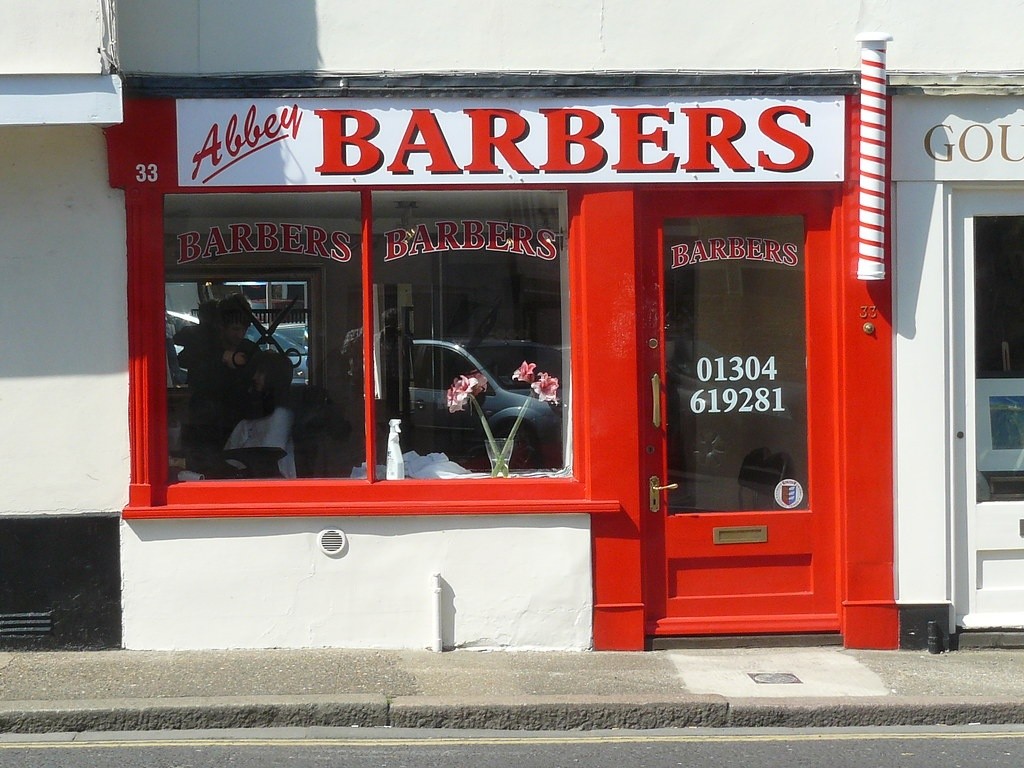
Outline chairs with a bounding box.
[738,447,791,511]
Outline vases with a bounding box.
[485,438,514,478]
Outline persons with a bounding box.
[176,294,262,480]
[221,351,298,478]
[341,308,414,464]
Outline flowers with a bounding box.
[446,360,559,440]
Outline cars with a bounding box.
[163,307,311,388]
[659,332,809,484]
[408,335,562,470]
